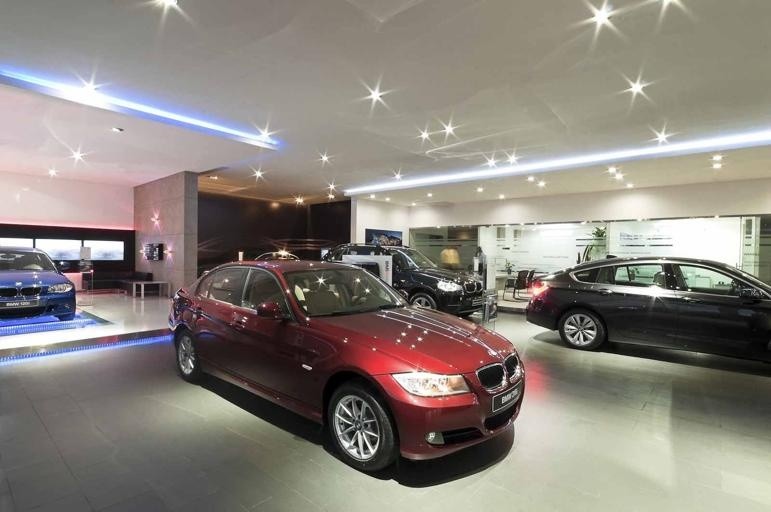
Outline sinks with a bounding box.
[82,271,159,297]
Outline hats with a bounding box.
[526,255,771,366]
[0,246,76,322]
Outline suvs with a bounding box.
[252,249,320,262]
[320,244,484,315]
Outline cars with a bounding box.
[0,246,76,322]
[169,258,525,478]
[526,255,771,366]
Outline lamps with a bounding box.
[308,274,339,316]
[249,273,282,309]
[502,269,535,302]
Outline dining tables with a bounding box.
[505,262,516,274]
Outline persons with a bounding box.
[474,247,487,275]
[440,245,460,271]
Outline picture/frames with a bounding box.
[496,272,547,296]
